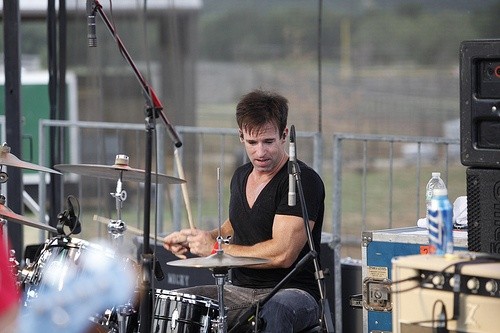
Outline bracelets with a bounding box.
[211,242,218,254]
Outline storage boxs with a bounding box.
[317,226,468,333]
[391,251,500,333]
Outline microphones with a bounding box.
[66,200,81,234]
[437,305,447,333]
[87,0,97,47]
[288,126,296,206]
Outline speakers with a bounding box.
[465,167,500,256]
[459,39,500,170]
[391,250,500,333]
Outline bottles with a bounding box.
[426,173,446,229]
[428,188,453,253]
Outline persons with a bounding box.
[0,225,21,333]
[163,90,326,333]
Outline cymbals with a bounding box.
[0,204,57,233]
[0,153,63,176]
[54,163,187,185]
[166,253,271,268]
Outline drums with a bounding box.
[140,288,227,333]
[20,237,140,333]
